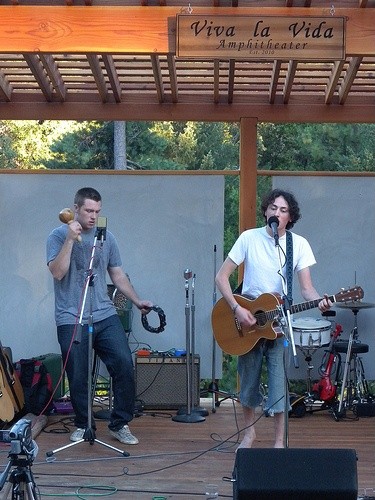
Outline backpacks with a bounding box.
[13,358,57,416]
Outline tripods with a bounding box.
[0,440,42,500]
[200,245,237,413]
[46,226,130,457]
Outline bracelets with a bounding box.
[233,304,240,314]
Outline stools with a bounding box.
[332,342,371,410]
[94,329,132,419]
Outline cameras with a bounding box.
[0,418,32,446]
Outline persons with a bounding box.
[215,189,334,454]
[46,187,153,445]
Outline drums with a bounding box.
[287,316,334,355]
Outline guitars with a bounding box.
[313,321,342,402]
[210,286,366,357]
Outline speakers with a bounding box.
[232,448,359,500]
[135,354,200,409]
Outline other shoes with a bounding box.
[70,427,85,441]
[110,425,139,445]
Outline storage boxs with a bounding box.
[107,284,132,330]
[36,353,64,398]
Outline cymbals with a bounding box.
[335,300,373,312]
[139,306,167,335]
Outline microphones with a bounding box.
[96,216,107,246]
[268,216,279,247]
